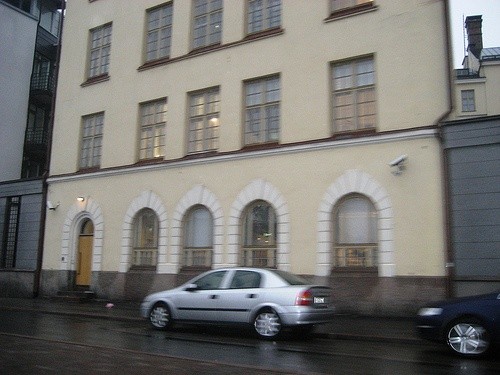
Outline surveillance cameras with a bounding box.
[388,154,408,167]
[46,200,55,211]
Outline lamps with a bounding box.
[76,196,84,202]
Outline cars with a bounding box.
[140,266,334,340]
[416,290,499,358]
